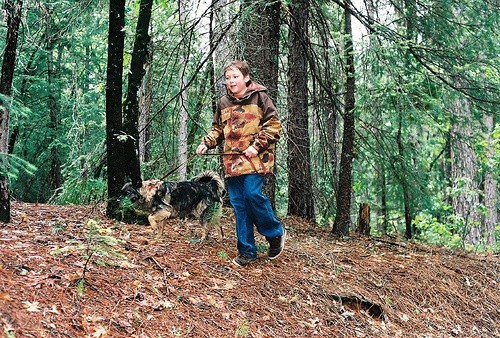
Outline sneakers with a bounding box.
[267,229,287,259]
[232,254,257,267]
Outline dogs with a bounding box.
[117,169,227,244]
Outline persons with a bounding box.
[195,61,286,266]
